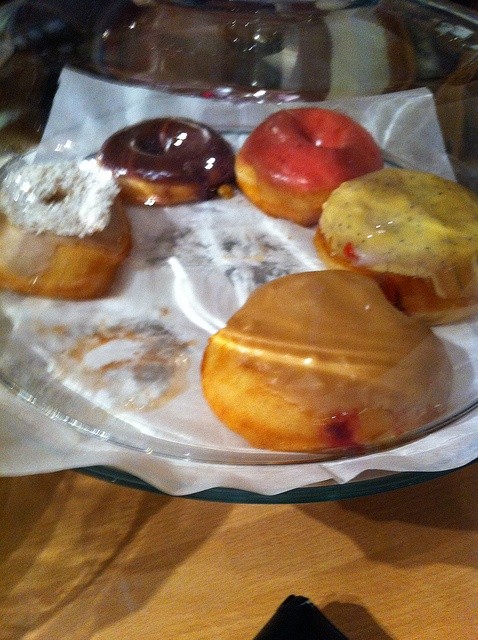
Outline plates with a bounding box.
[70,462,478,507]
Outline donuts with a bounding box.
[0,156,133,299]
[232,108,386,224]
[101,116,232,206]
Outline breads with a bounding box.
[200,270,452,450]
[317,167,477,323]
